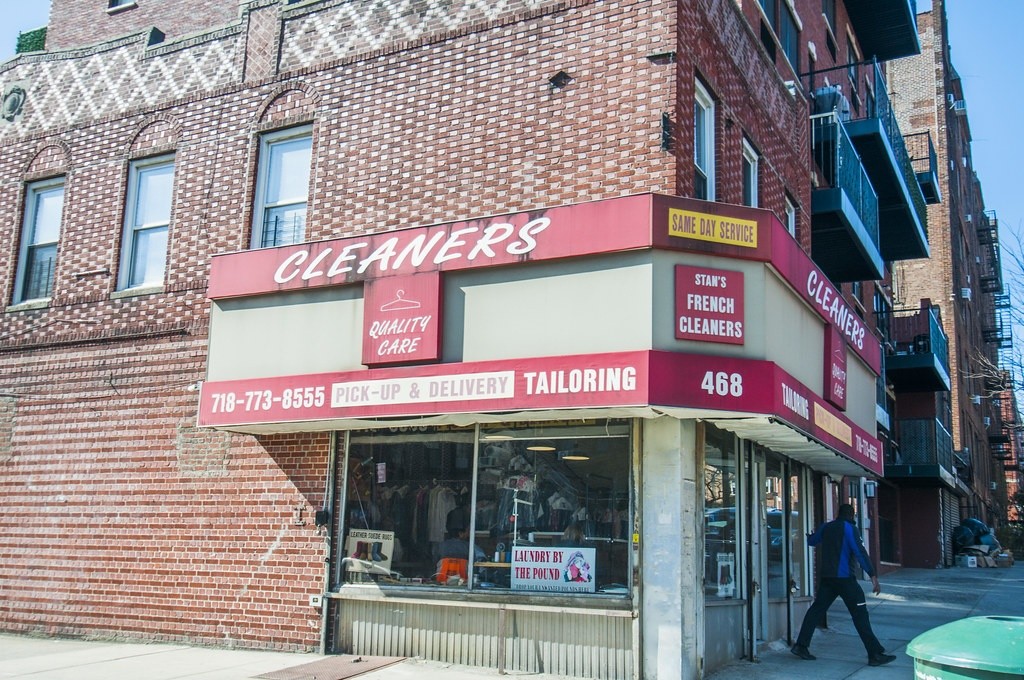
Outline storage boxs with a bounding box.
[954,555,978,568]
[995,554,1012,568]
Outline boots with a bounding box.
[360,542,368,560]
[375,542,388,560]
[367,542,373,561]
[373,543,382,561]
[351,541,362,558]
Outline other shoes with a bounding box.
[868,653,896,666]
[791,644,816,660]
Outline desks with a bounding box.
[475,561,512,590]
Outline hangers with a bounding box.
[378,478,470,495]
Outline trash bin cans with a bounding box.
[904,614,1024,680]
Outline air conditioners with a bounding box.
[975,257,981,264]
[961,287,972,300]
[966,275,971,284]
[984,417,991,426]
[948,94,954,103]
[962,158,967,168]
[972,395,981,405]
[955,99,966,116]
[950,160,954,171]
[991,481,997,489]
[965,215,973,223]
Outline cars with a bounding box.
[706,507,798,561]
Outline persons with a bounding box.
[554,523,595,548]
[434,508,488,580]
[790,504,898,668]
